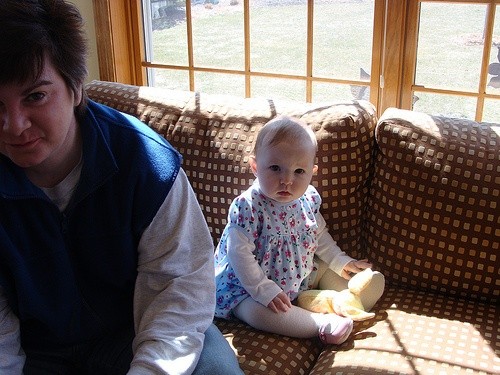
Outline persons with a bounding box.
[214,114,385,345]
[0,0,245,375]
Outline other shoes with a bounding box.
[319,313,353,345]
[347,268,385,314]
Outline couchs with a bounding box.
[84,78,500,375]
